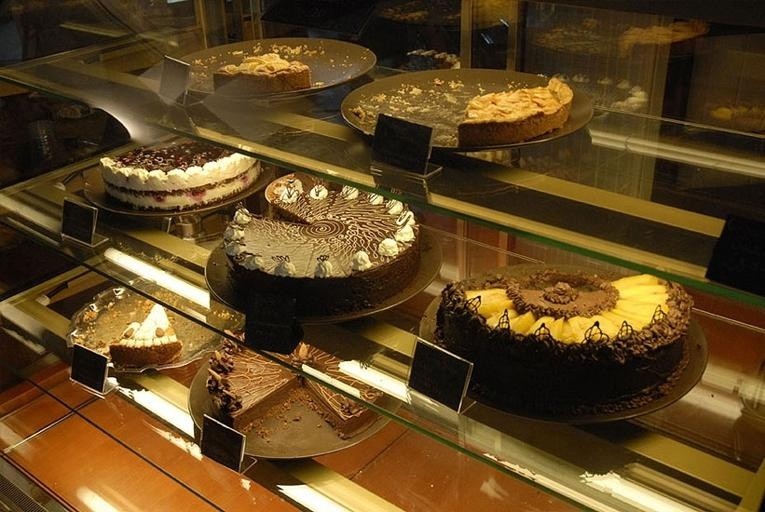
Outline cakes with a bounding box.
[438,267,694,414]
[100,143,264,210]
[223,173,422,316]
[206,326,390,441]
[109,301,183,368]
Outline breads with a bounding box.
[213,52,312,93]
[459,77,574,149]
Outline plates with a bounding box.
[340,67,594,153]
[203,226,443,326]
[65,284,224,373]
[419,293,711,427]
[83,160,277,219]
[178,37,378,106]
[188,358,404,461]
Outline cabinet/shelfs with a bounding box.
[0,0,765,512]
